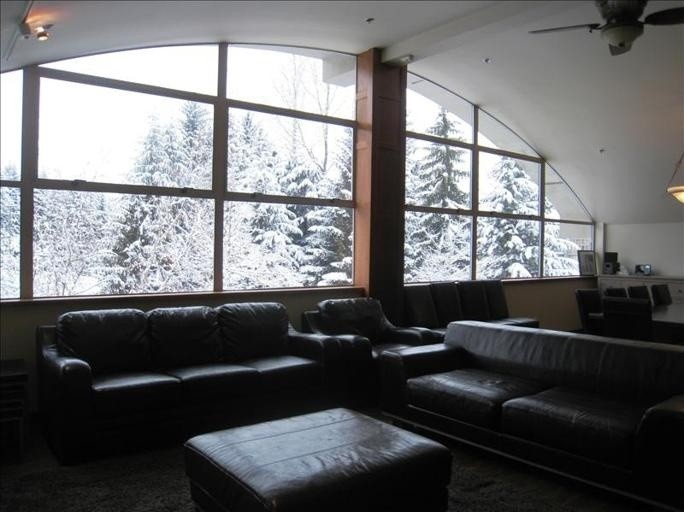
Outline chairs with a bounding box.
[572,280,673,345]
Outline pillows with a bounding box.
[55,308,151,374]
[314,296,384,346]
[213,301,289,363]
[145,303,225,373]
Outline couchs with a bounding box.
[32,298,348,471]
[401,278,541,343]
[391,318,681,510]
[296,295,425,416]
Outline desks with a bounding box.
[651,300,684,346]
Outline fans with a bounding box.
[527,0,684,57]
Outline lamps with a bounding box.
[600,13,645,50]
[663,149,684,205]
[18,22,52,42]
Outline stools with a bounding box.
[181,405,455,511]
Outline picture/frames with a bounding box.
[577,248,596,277]
